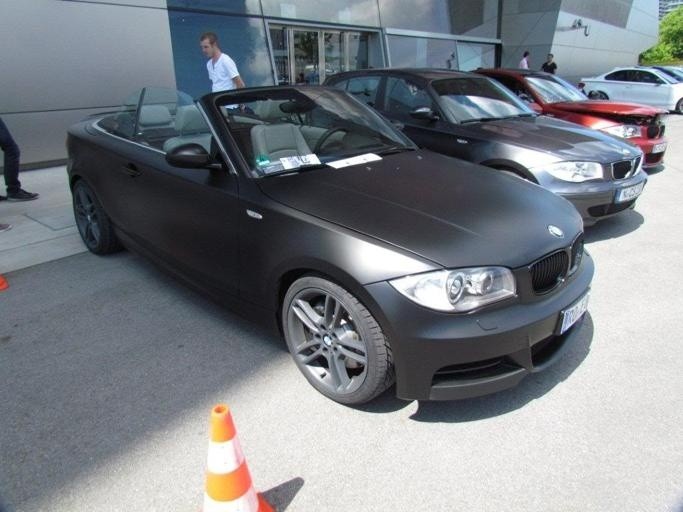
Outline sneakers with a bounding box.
[6,186,40,201]
[0,223,11,232]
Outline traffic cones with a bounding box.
[200,400,281,512]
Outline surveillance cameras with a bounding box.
[585,25,590,36]
[572,19,578,27]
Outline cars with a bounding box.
[320,62,651,234]
[652,65,683,83]
[570,67,683,116]
[470,65,673,171]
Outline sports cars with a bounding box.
[58,75,603,414]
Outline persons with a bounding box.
[198,31,245,154]
[518,51,530,70]
[539,54,557,75]
[0,115,39,235]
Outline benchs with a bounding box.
[135,98,322,173]
